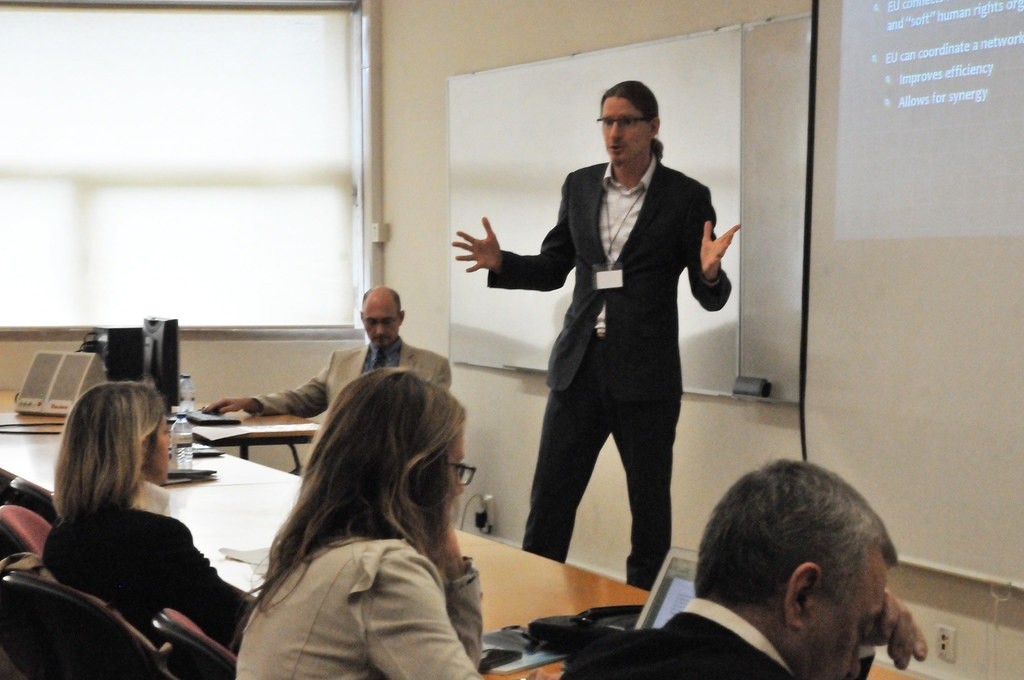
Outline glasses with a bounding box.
[450,463,476,485]
[362,314,400,327]
[597,116,652,129]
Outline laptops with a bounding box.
[635,547,700,631]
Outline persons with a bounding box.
[202,286,452,416]
[41,381,256,650]
[452,81,742,593]
[558,457,927,679]
[237,366,486,679]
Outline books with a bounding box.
[481,626,565,674]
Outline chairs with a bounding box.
[0,571,180,680]
[0,505,52,556]
[149,609,236,680]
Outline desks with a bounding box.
[0,413,923,680]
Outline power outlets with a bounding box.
[935,623,958,664]
[482,495,494,526]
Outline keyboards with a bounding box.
[186,413,241,425]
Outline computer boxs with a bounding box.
[93,326,143,381]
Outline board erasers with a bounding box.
[733,376,772,398]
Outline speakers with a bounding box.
[15,350,108,417]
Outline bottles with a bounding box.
[178,374,195,413]
[171,414,194,469]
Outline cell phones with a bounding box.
[478,649,522,670]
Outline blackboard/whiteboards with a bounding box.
[449,7,814,404]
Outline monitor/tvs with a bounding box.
[140,314,185,423]
